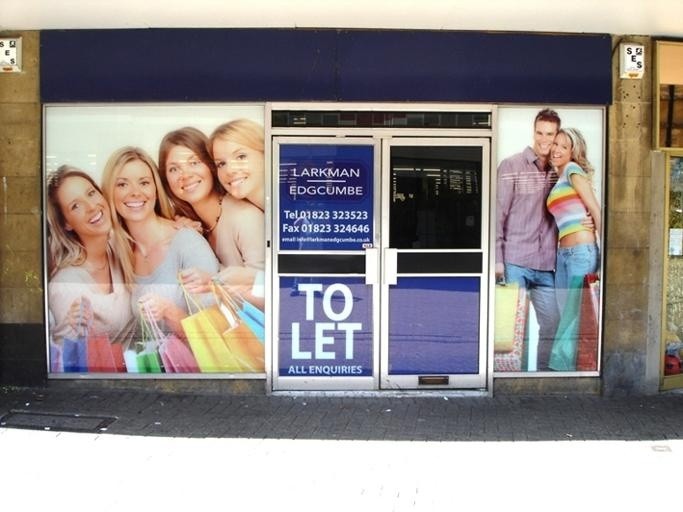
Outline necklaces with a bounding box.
[205,197,222,232]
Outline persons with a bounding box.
[207,119,265,212]
[102,146,221,345]
[48,165,142,345]
[158,125,265,312]
[494,106,598,372]
[546,128,600,370]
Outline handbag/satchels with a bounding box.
[494,282,529,371]
[50,301,265,373]
[547,273,600,372]
